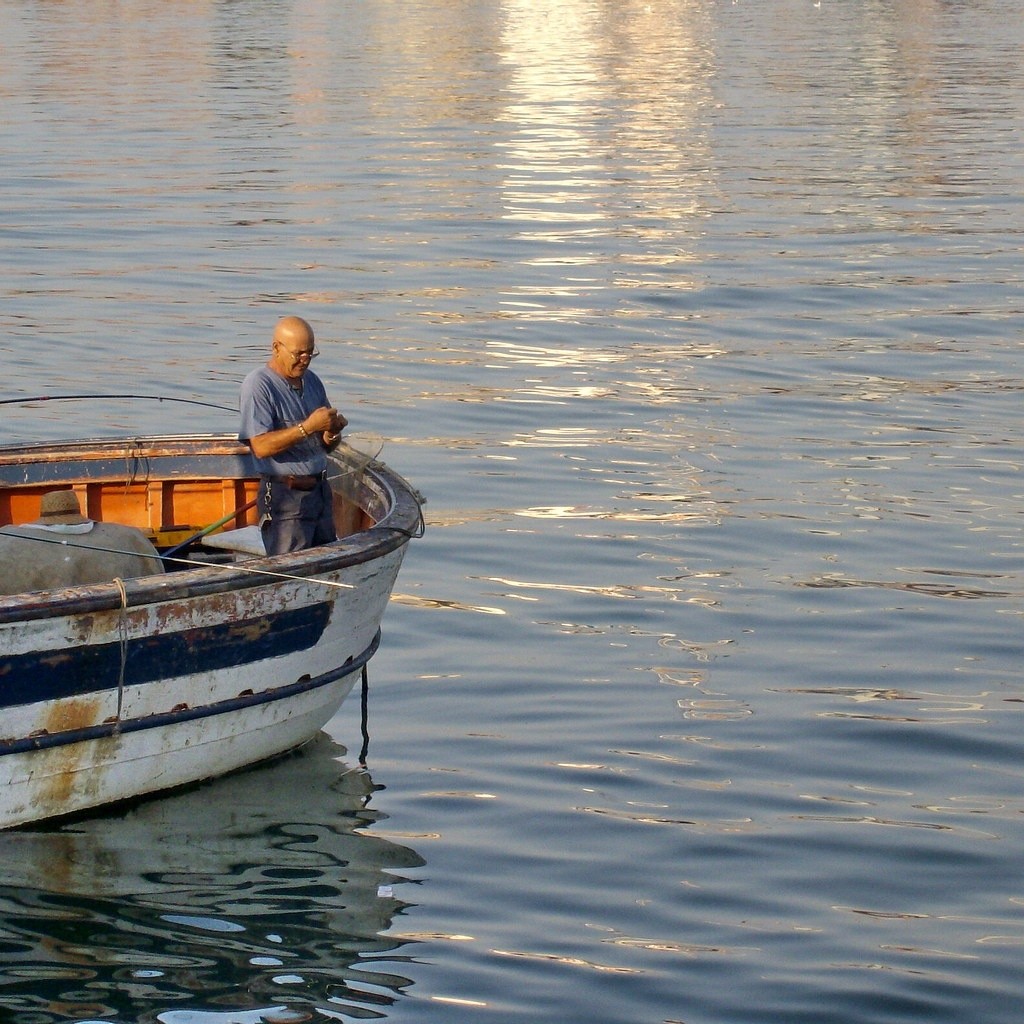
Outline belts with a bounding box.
[260,473,327,489]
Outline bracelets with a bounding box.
[298,424,309,438]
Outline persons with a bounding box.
[238,315,348,557]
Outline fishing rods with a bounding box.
[1,393,240,415]
[2,531,359,594]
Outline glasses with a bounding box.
[278,340,320,362]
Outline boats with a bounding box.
[0,393,427,833]
